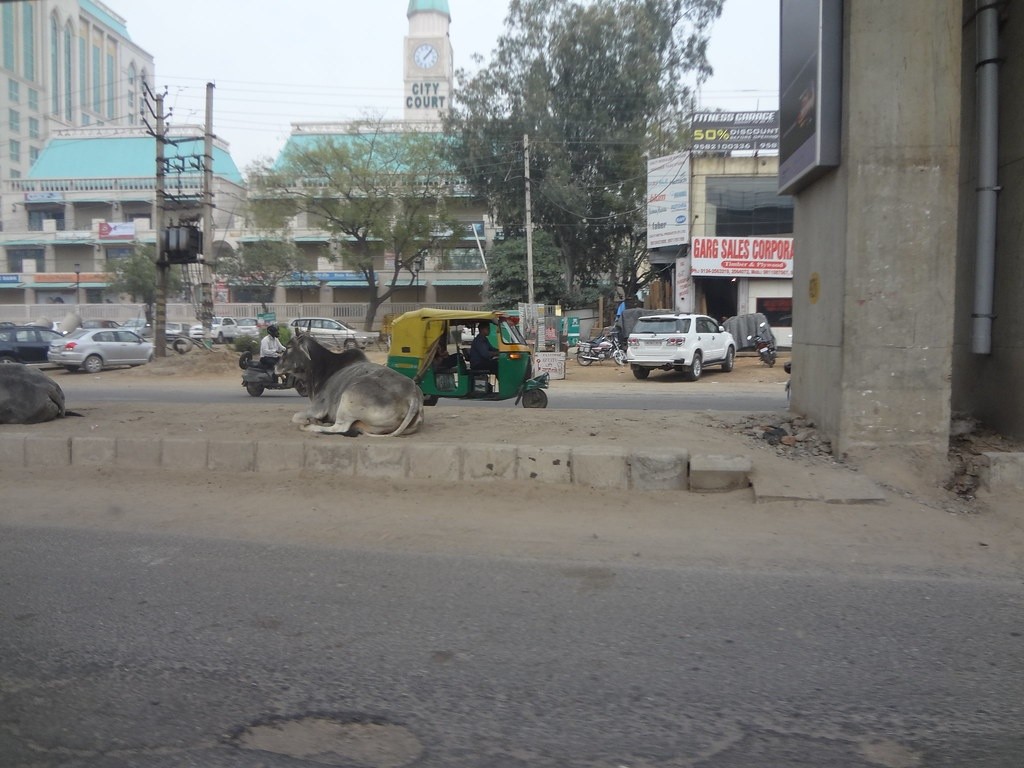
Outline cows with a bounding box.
[0,362,86,425]
[271,318,425,438]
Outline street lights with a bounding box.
[75,263,81,305]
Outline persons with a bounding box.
[433,321,499,381]
[259,323,288,385]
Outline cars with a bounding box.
[768,314,793,348]
[258,319,281,331]
[234,317,261,341]
[46,325,157,374]
[0,320,71,364]
[288,316,380,351]
[118,319,154,340]
[164,321,193,343]
[79,318,122,329]
[188,317,239,345]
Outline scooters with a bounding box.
[238,348,309,398]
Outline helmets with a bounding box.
[267,325,279,335]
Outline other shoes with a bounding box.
[278,376,283,385]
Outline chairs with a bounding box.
[435,366,459,373]
[461,347,491,394]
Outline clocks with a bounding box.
[414,44,438,69]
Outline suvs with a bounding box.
[626,312,736,382]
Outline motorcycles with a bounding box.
[575,321,627,368]
[747,321,778,368]
[387,308,551,408]
